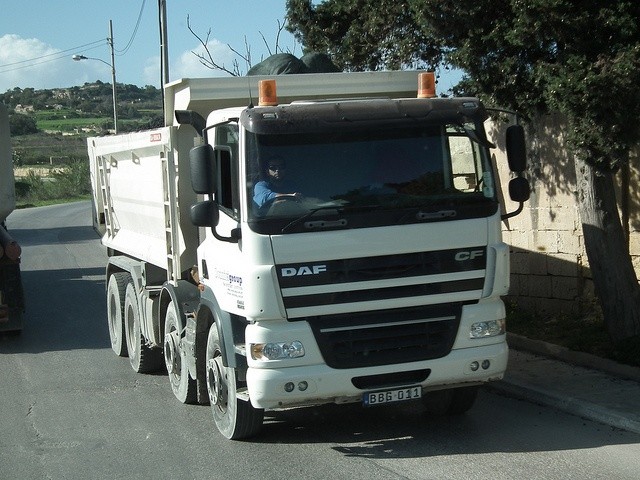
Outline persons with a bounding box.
[252,155,305,206]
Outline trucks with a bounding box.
[77,68,532,446]
